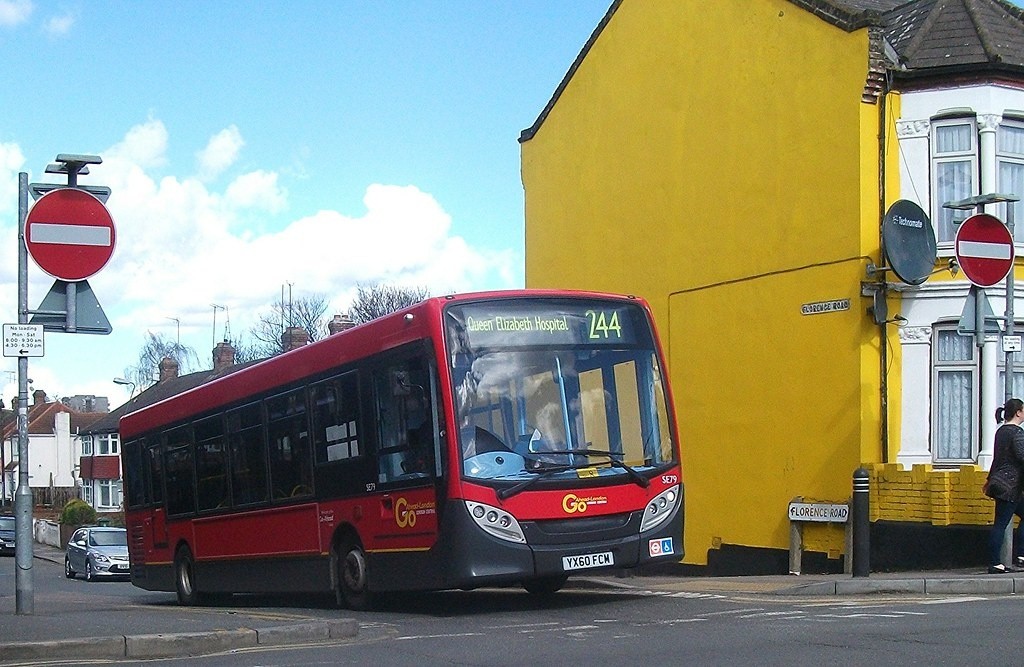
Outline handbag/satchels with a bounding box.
[985,464,1020,502]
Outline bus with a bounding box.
[119,290,686,611]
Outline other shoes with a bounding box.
[1016,558,1024,566]
[988,566,1014,573]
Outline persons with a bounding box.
[986,399,1024,574]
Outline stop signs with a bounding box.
[954,213,1015,288]
[23,188,117,281]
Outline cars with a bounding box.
[0,515,15,557]
[65,526,130,582]
